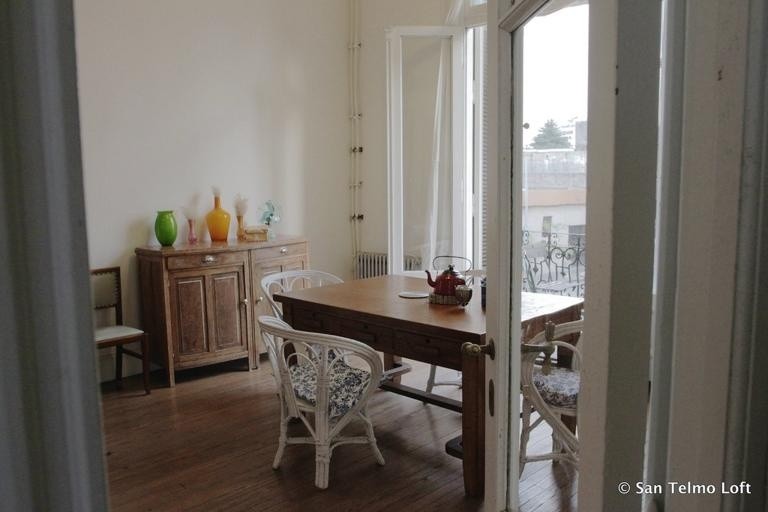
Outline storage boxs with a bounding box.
[245,225,270,243]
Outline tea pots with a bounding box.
[423,255,475,296]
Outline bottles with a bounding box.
[154,192,246,247]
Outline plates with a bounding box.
[397,290,434,300]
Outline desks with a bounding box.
[273,274,585,499]
[536,280,584,293]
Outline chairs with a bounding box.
[89,266,152,397]
[260,268,383,424]
[254,314,388,491]
[517,293,658,482]
[420,269,489,406]
[522,253,535,293]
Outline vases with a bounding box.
[236,215,246,240]
[206,196,231,242]
[154,209,179,246]
[187,218,198,244]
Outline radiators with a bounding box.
[353,250,421,280]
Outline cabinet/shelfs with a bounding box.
[134,235,313,390]
